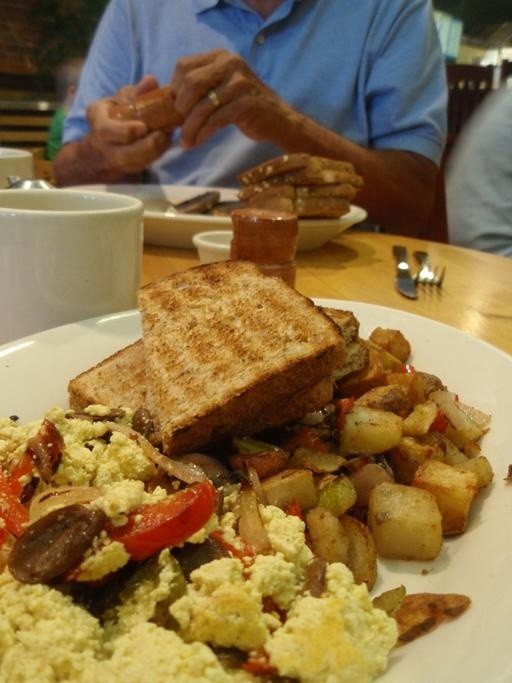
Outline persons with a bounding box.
[45,55,87,160]
[42,1,451,245]
[439,74,511,258]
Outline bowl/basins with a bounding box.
[190,229,231,266]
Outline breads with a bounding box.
[236,151,363,220]
[68,260,370,451]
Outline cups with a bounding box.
[0,146,33,188]
[0,189,145,343]
[228,206,300,294]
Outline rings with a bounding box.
[207,88,220,107]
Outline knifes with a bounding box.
[391,243,418,300]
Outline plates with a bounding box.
[59,179,369,256]
[1,298,512,682]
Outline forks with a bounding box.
[410,249,447,289]
